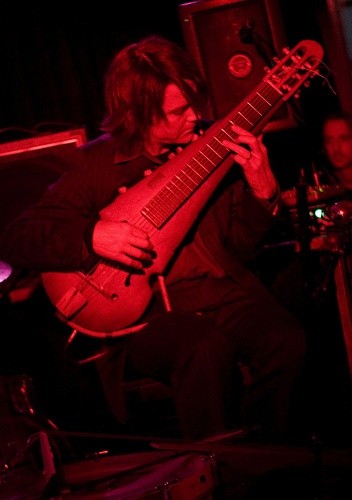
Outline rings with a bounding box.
[245,153,253,160]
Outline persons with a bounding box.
[0,32,312,477]
[291,112,352,370]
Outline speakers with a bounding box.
[181,0,300,132]
